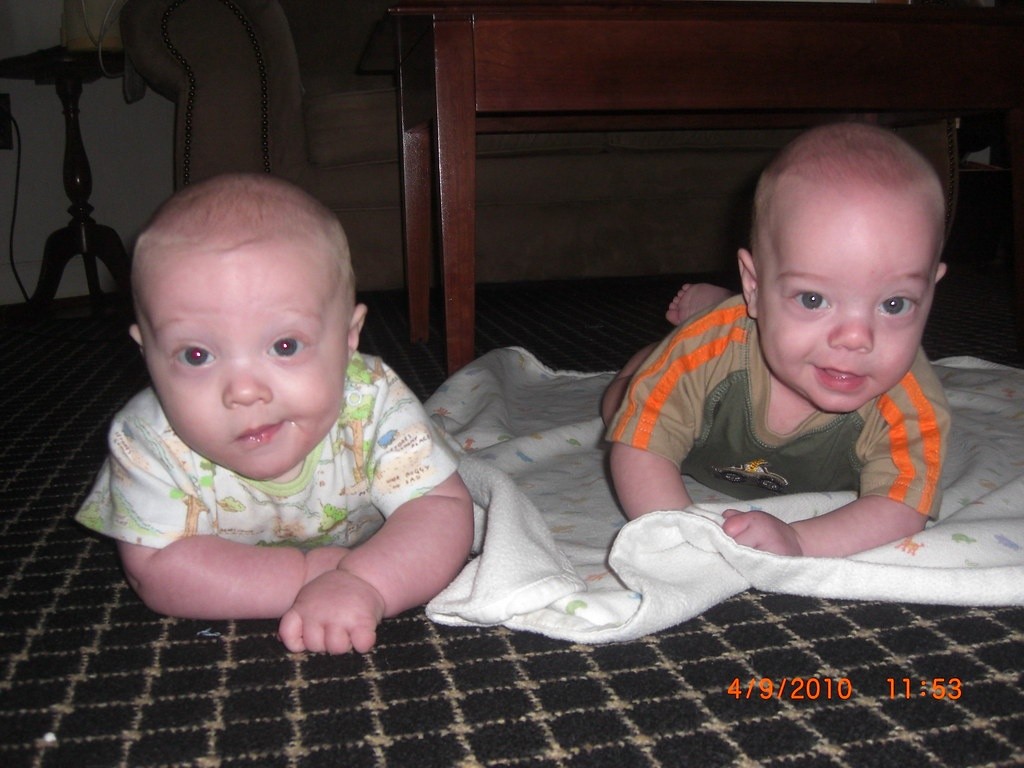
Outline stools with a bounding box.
[0,48,132,312]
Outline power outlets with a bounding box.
[0,93,14,150]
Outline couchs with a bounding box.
[120,0,961,297]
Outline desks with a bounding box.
[356,0,1024,376]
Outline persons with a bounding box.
[599,125,953,557]
[77,175,476,654]
